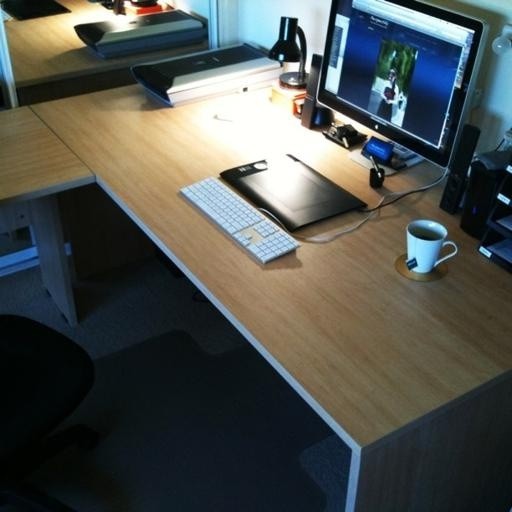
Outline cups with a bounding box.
[406,219,458,273]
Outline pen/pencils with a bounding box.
[370,155,382,178]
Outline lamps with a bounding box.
[268,16,309,88]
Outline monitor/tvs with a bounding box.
[315,1,490,178]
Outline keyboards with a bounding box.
[179,175,302,267]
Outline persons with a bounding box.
[375,67,400,124]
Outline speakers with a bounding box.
[301,52,323,130]
[439,123,482,217]
